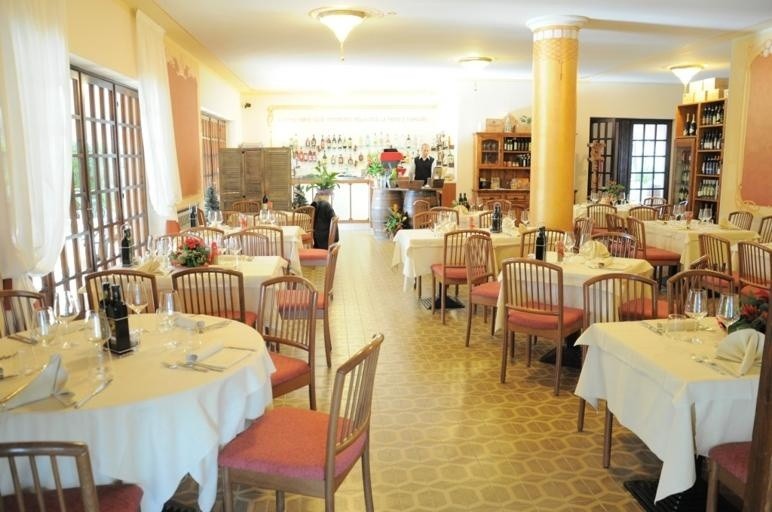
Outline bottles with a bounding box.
[504,138,530,167]
[676,148,691,202]
[683,112,691,135]
[505,118,511,132]
[287,128,455,177]
[697,104,725,200]
[689,112,696,136]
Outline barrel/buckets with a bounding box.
[403,189,437,229]
[370,187,404,241]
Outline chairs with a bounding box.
[171,269,258,327]
[2,288,46,338]
[85,269,159,312]
[151,199,316,288]
[280,215,341,368]
[259,275,317,411]
[217,334,384,511]
[391,199,772,512]
[2,441,144,512]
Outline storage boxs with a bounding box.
[485,118,505,132]
[682,76,728,103]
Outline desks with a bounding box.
[0,312,277,510]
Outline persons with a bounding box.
[408,143,436,186]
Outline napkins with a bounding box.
[185,337,252,373]
[1,351,77,408]
[167,315,227,332]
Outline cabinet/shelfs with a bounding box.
[665,99,728,223]
[471,132,532,211]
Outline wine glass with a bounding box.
[534,218,613,271]
[640,288,741,379]
[426,190,531,241]
[580,189,633,210]
[656,200,714,232]
[0,281,208,408]
[113,219,244,273]
[190,192,277,238]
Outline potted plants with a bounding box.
[312,161,340,195]
[386,167,397,188]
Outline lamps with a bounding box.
[310,8,369,60]
[669,63,705,93]
